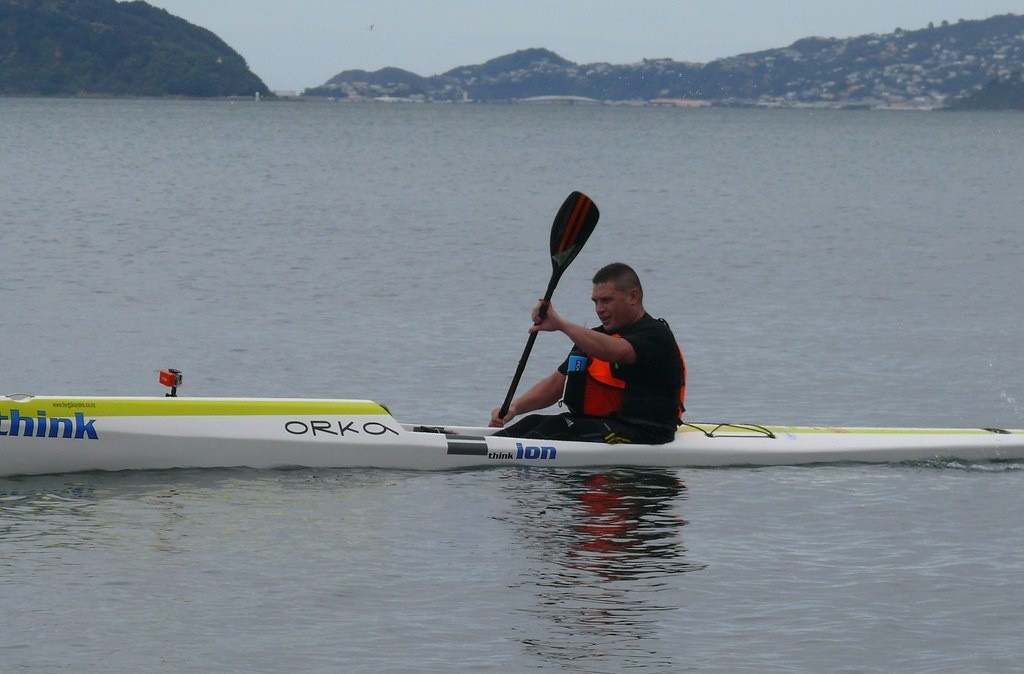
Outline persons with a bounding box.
[486,262,688,445]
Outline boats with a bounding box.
[1,366,1023,482]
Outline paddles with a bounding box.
[498,190,602,423]
